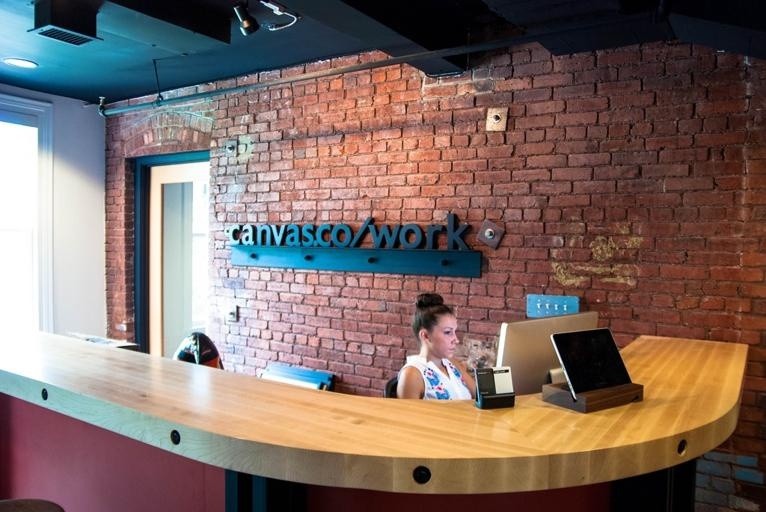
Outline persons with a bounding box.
[397,292,478,401]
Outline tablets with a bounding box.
[550,326,632,400]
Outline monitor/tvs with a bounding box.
[495,310,598,393]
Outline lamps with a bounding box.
[232,1,301,38]
